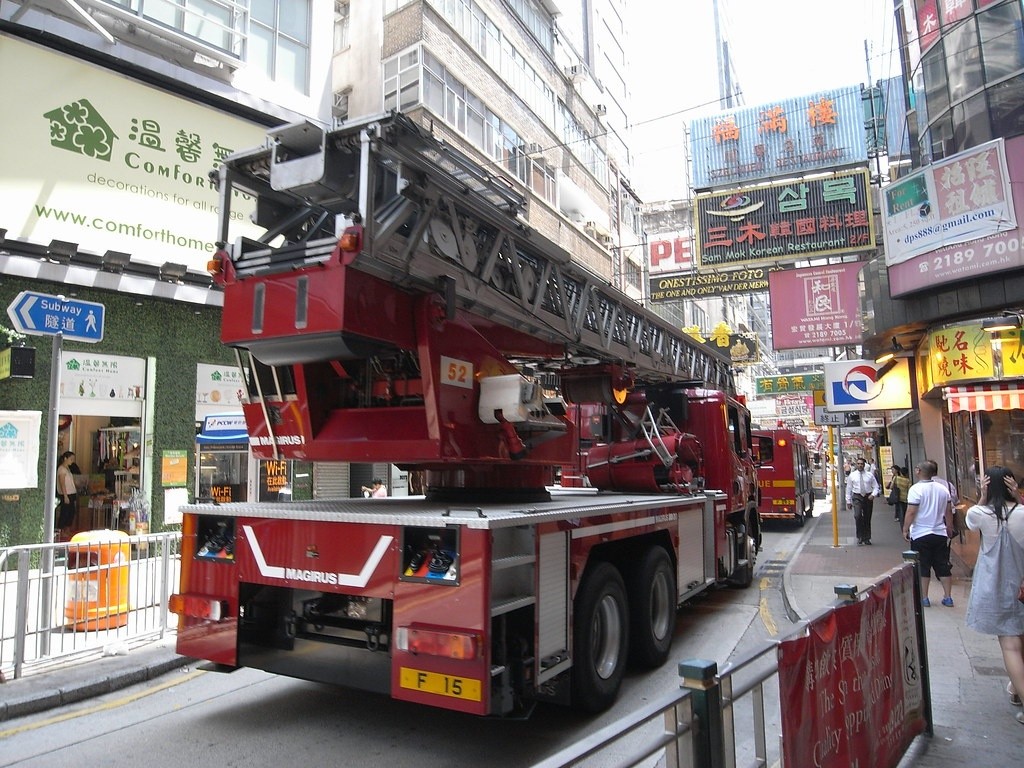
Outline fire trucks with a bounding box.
[168,110,775,725]
[730,419,820,528]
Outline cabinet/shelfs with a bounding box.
[101,425,142,528]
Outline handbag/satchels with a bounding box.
[888,476,901,506]
[1018,580,1024,603]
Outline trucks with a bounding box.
[807,447,830,500]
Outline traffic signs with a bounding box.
[7,290,106,344]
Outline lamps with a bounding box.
[875,347,917,364]
[980,309,1023,332]
[46,239,79,265]
[159,261,187,285]
[100,250,131,274]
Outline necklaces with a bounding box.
[62,464,72,476]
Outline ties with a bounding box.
[860,472,866,497]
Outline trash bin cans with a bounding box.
[63,528,129,631]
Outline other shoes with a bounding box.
[942,597,953,606]
[895,518,899,522]
[864,539,871,545]
[857,537,863,544]
[922,598,930,607]
[1007,681,1022,705]
[1016,712,1024,723]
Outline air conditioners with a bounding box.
[593,104,606,115]
[566,63,590,83]
[634,206,644,214]
[622,193,632,201]
[527,141,544,159]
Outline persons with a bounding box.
[843,457,879,546]
[965,466,1024,724]
[57,451,77,538]
[361,477,387,499]
[885,460,961,607]
